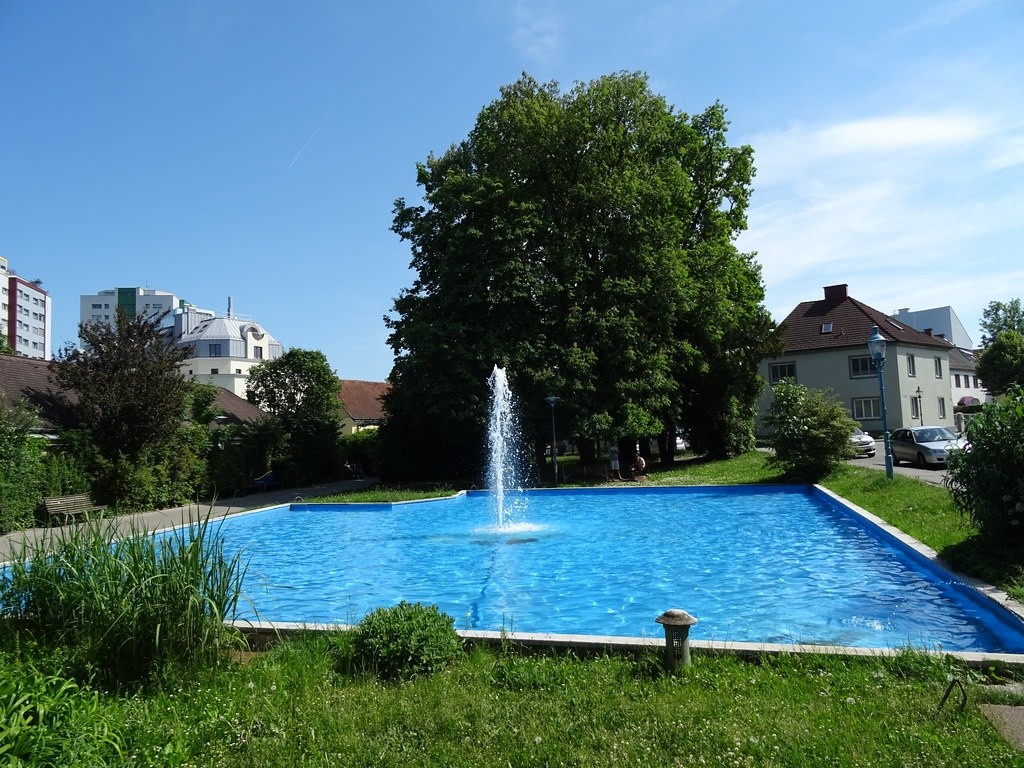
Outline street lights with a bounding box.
[866,325,894,480]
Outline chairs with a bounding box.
[901,433,908,440]
[931,431,939,440]
[910,433,918,439]
[919,432,927,441]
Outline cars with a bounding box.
[886,426,971,469]
[957,396,980,405]
[848,428,876,457]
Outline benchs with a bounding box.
[344,463,366,480]
[44,493,109,519]
